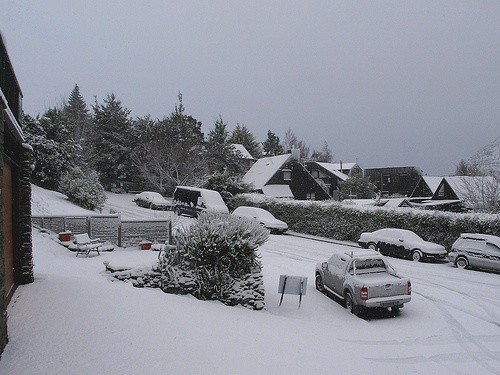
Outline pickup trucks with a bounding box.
[315,247,412,315]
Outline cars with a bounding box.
[358,227,447,262]
[448,233,500,273]
[232,206,288,235]
[134,191,172,211]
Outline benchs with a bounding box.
[74,232,102,256]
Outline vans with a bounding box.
[172,186,229,219]
